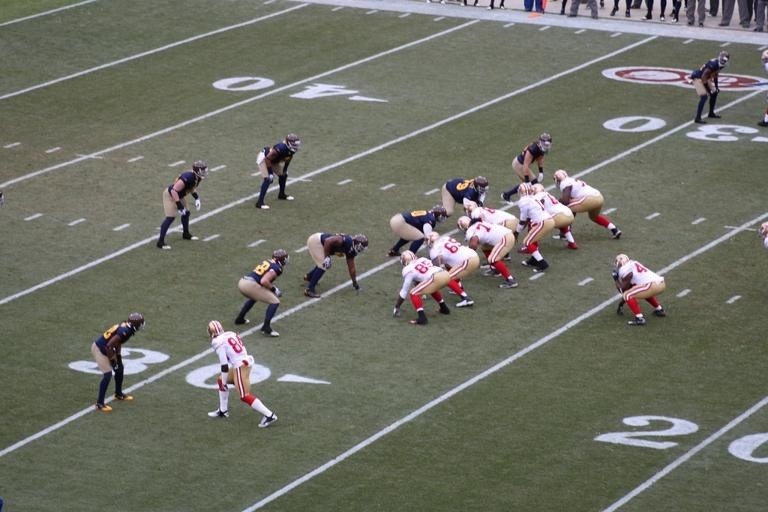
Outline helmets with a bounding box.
[271,249,290,268]
[718,51,730,67]
[286,134,303,151]
[350,234,370,255]
[616,255,630,271]
[400,176,489,267]
[207,321,226,338]
[515,132,569,196]
[192,161,208,179]
[128,312,146,330]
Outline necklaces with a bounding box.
[254,133,300,209]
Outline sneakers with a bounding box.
[613,270,620,281]
[254,203,271,210]
[552,233,577,249]
[388,249,400,256]
[304,289,319,295]
[113,394,133,402]
[182,233,197,240]
[156,242,170,249]
[259,413,278,427]
[259,328,278,337]
[653,307,665,317]
[94,402,113,414]
[483,246,547,288]
[629,318,647,325]
[695,117,705,124]
[235,319,249,324]
[208,409,230,418]
[425,0,504,11]
[409,283,473,324]
[707,113,721,119]
[610,229,621,237]
[611,4,712,28]
[278,193,293,199]
[303,273,313,281]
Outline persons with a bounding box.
[456,0,767,36]
[756,50,768,129]
[686,49,730,125]
[90,312,146,412]
[206,319,277,427]
[302,232,369,298]
[155,161,209,250]
[233,248,289,336]
[612,253,668,325]
[499,132,553,204]
[382,168,621,324]
[759,220,767,248]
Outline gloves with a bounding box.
[268,174,274,184]
[109,356,118,371]
[322,257,333,269]
[195,199,201,211]
[271,285,282,296]
[617,304,623,315]
[221,383,227,391]
[177,206,187,215]
[353,282,360,290]
